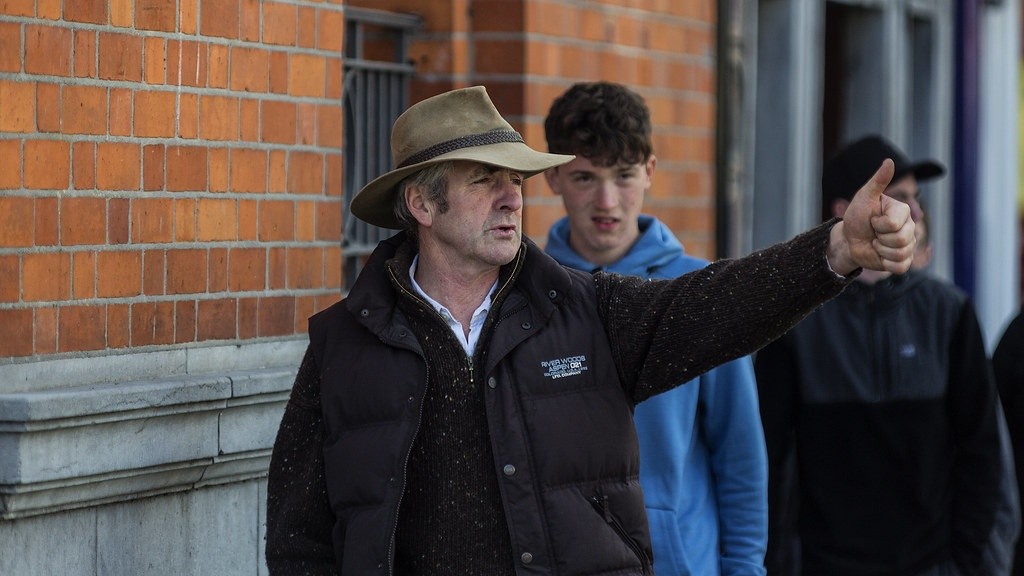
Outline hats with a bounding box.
[822,132,943,199]
[347,81,573,230]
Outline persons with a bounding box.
[754,129,1005,576]
[262,79,920,576]
[896,207,1022,575]
[988,302,1023,576]
[534,77,774,576]
